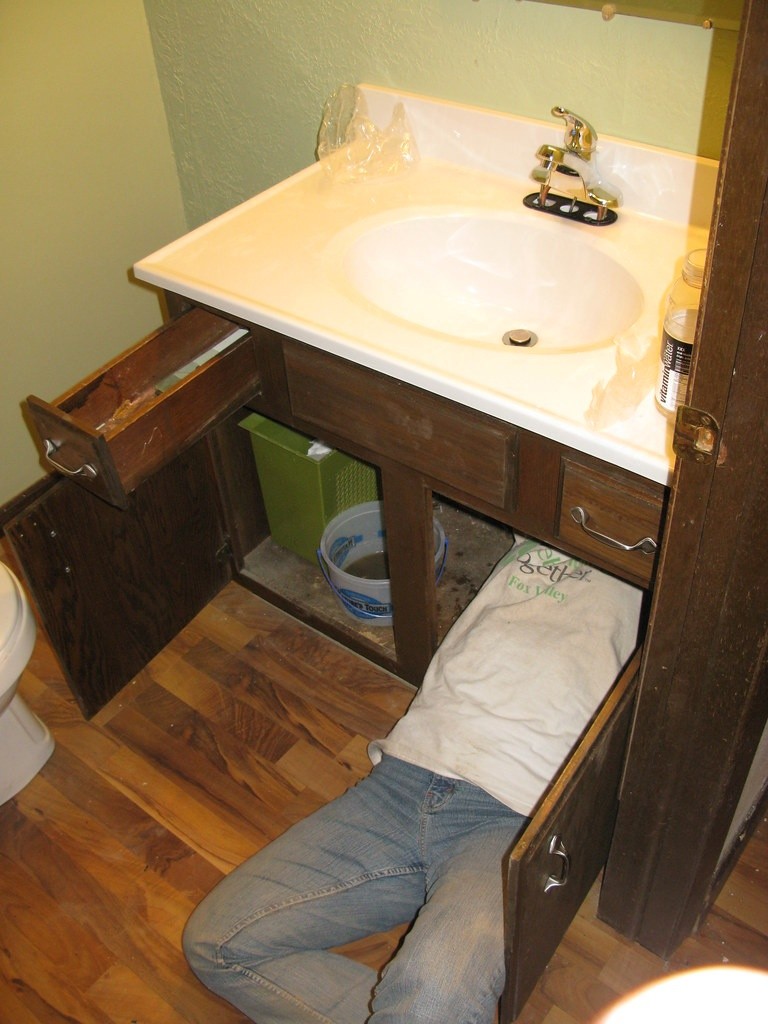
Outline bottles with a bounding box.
[659,249,705,417]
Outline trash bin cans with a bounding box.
[237,411,382,565]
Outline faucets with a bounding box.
[523,106,619,220]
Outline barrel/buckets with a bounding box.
[315,501,447,625]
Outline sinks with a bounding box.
[129,83,721,483]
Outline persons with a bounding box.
[182,531,644,1024]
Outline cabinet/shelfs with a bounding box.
[0,289,671,1024]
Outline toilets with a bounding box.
[0,558,58,808]
